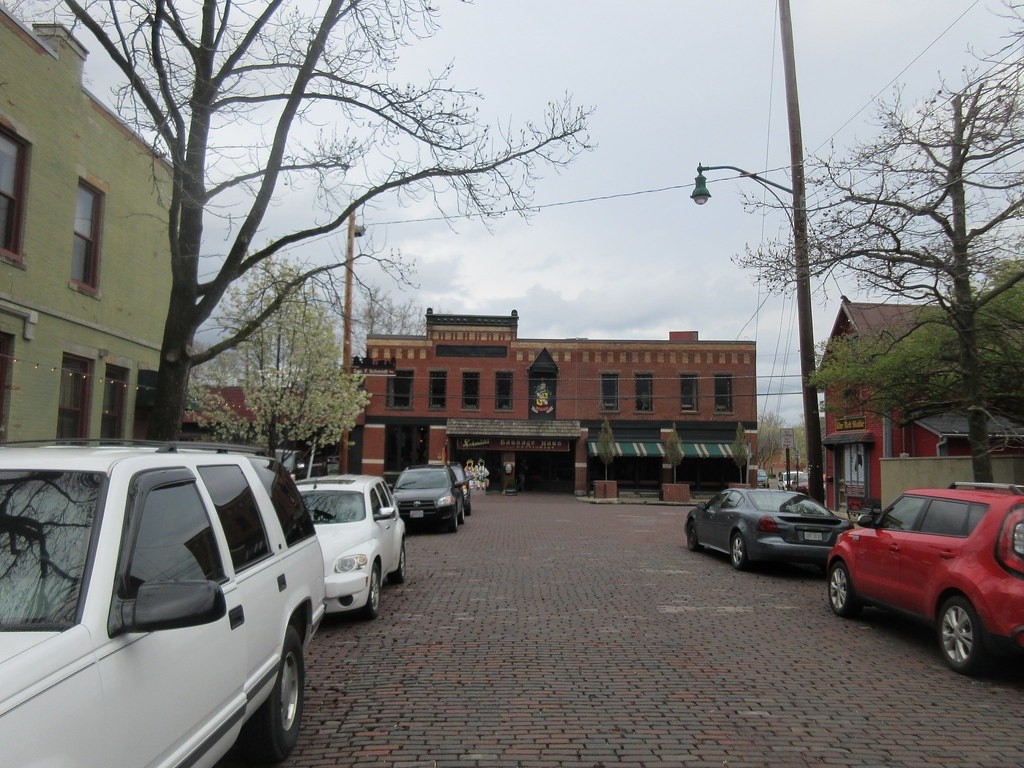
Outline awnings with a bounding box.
[587,442,746,458]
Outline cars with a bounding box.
[791,474,826,494]
[756,469,769,488]
[685,488,855,571]
[295,474,406,618]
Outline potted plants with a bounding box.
[594,415,618,498]
[726,422,751,489]
[659,421,691,502]
[504,478,518,496]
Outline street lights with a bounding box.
[691,166,825,504]
[339,224,367,474]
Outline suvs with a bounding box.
[0,439,327,768]
[828,479,1024,671]
[273,449,308,482]
[389,464,466,533]
[778,471,803,490]
[429,461,474,515]
